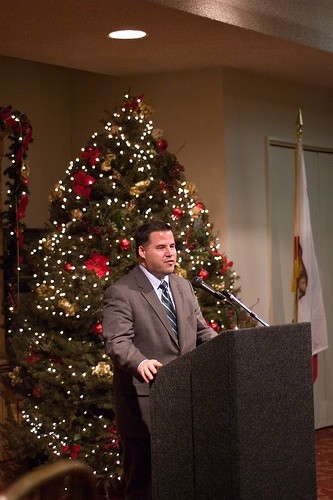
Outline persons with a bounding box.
[102,221,221,499]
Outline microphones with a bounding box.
[222,289,270,327]
[192,276,233,305]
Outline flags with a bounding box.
[293,134,329,381]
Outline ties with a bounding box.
[159,281,179,343]
